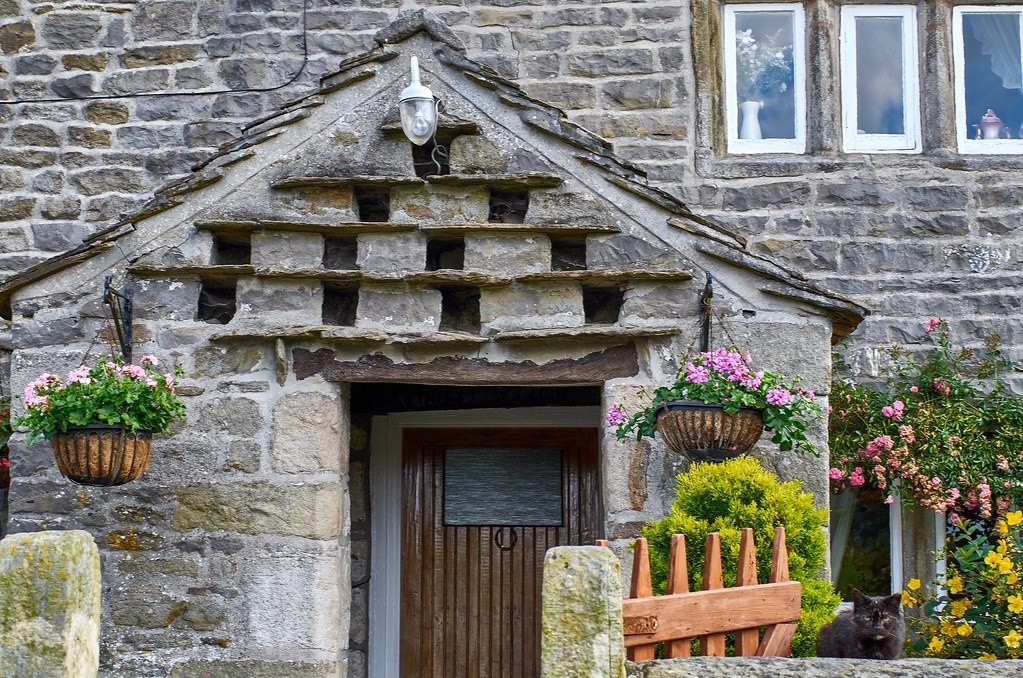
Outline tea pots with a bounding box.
[972,108,1012,139]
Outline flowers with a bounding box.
[607,345,826,459]
[735,27,792,106]
[831,317,1023,661]
[0,352,187,469]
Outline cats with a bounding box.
[815,588,906,660]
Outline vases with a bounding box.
[41,426,155,487]
[649,399,766,461]
[738,102,765,139]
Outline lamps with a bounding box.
[398,55,437,147]
